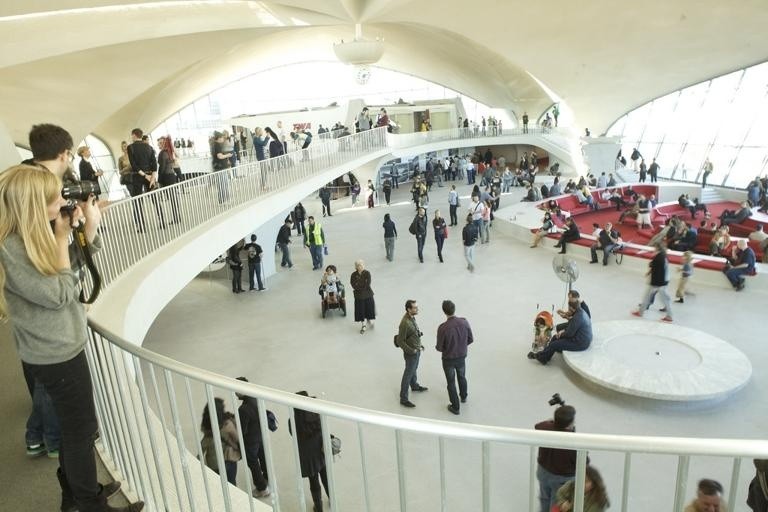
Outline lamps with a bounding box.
[332,24,386,66]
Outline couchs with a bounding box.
[538,186,659,217]
[652,204,700,226]
[688,228,765,261]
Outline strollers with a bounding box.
[529,301,560,357]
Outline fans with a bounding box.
[551,253,579,316]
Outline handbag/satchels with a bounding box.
[393,334,401,348]
[320,436,344,457]
[266,409,280,432]
[443,225,450,239]
[547,225,557,234]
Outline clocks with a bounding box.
[356,67,372,85]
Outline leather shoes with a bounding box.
[412,385,429,392]
[399,399,416,408]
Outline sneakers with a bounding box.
[252,487,270,498]
[46,432,101,459]
[103,501,145,512]
[25,443,47,456]
[60,480,122,512]
[448,404,460,415]
[461,398,466,403]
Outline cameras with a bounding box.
[417,330,424,338]
[63,179,101,203]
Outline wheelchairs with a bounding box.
[318,280,346,318]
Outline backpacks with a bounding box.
[197,433,227,475]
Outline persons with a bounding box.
[458,107,559,138]
[197,377,330,510]
[528,145,767,323]
[436,301,473,414]
[276,202,327,270]
[319,265,346,305]
[228,234,265,293]
[23,168,76,399]
[21,124,99,460]
[686,479,727,511]
[535,405,609,511]
[397,300,428,408]
[420,118,432,142]
[290,107,392,161]
[76,128,192,234]
[209,121,290,204]
[319,185,332,216]
[585,129,590,136]
[0,164,144,512]
[527,289,592,365]
[342,148,531,271]
[351,260,376,333]
